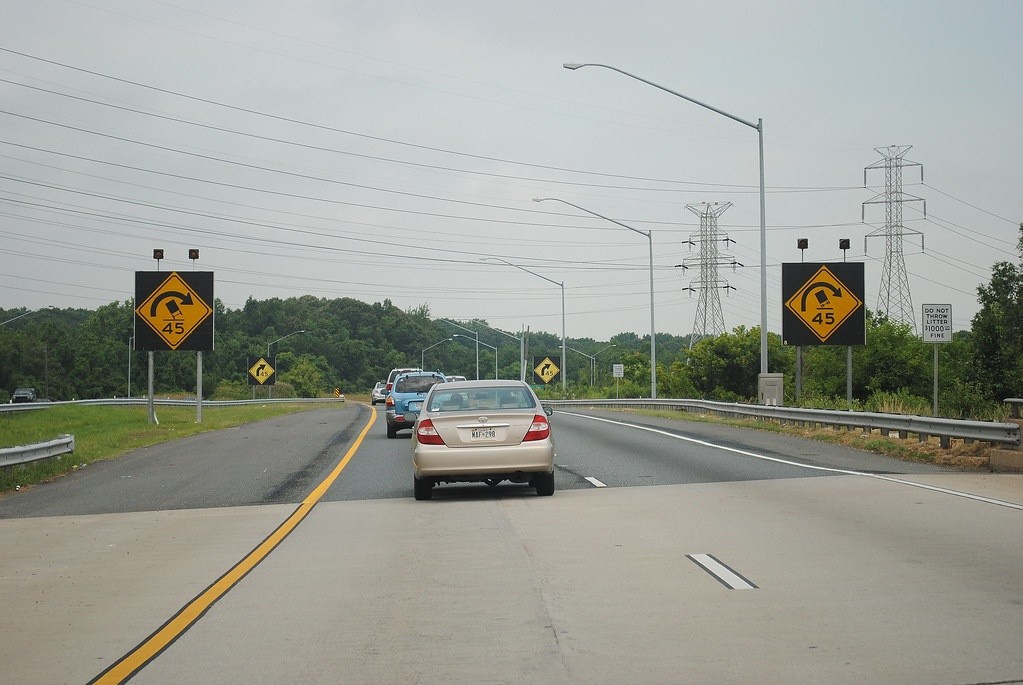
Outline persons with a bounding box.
[450,393,464,409]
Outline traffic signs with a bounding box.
[246,356,277,385]
[533,355,561,385]
[133,270,215,352]
[780,261,869,347]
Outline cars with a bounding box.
[403,379,555,501]
[369,382,387,405]
[380,367,471,439]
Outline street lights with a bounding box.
[591,344,617,387]
[479,257,567,400]
[452,334,498,401]
[562,60,770,372]
[422,338,453,371]
[267,330,305,357]
[532,196,658,400]
[467,317,525,381]
[557,345,596,387]
[429,315,479,381]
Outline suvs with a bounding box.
[11,387,37,403]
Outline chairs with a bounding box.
[500,396,521,409]
[439,400,462,411]
